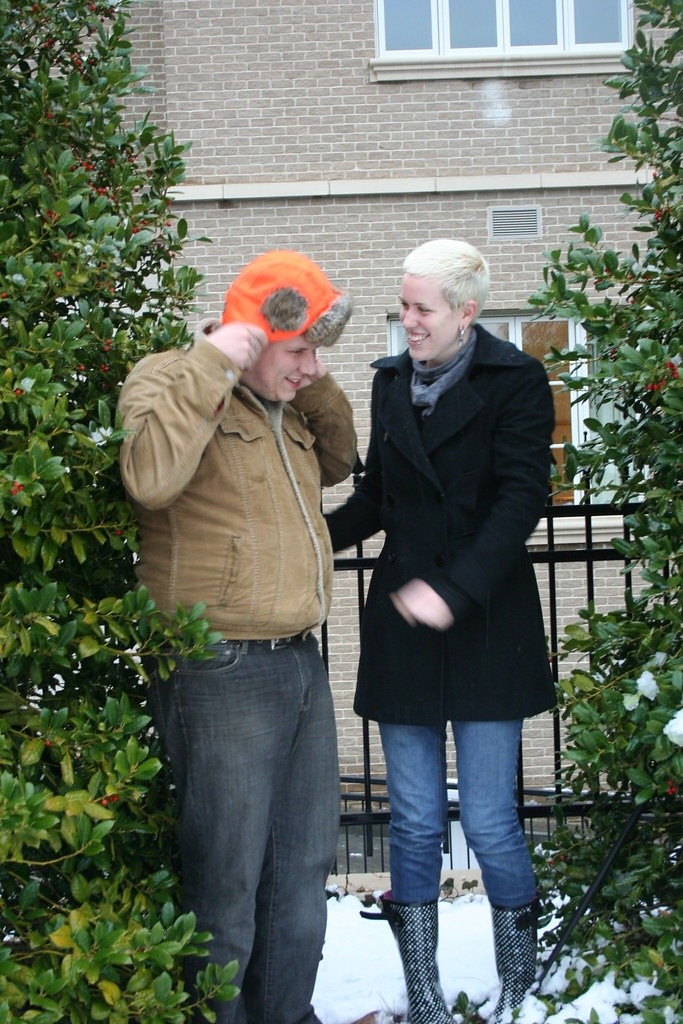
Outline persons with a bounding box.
[116,250,358,1024]
[325,239,556,1024]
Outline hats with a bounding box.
[222,250,352,347]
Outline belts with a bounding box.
[215,632,301,651]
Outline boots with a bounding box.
[488,894,538,1024]
[360,891,459,1024]
